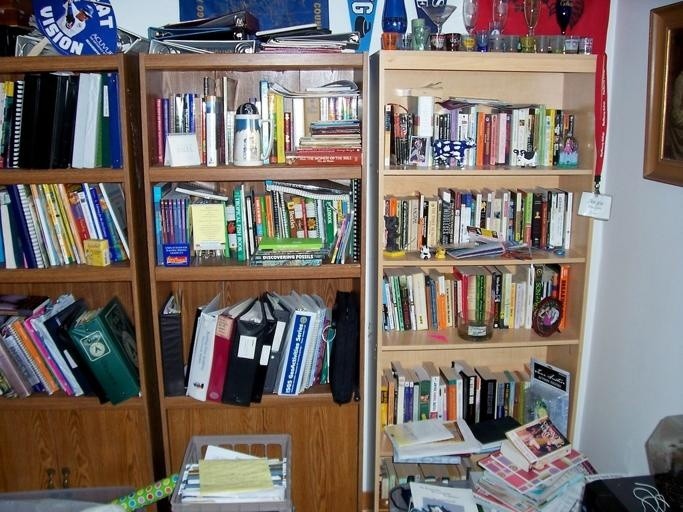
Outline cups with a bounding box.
[489,0,509,30]
[381,17,431,50]
[232,112,274,168]
[462,0,478,34]
[429,28,594,55]
[457,309,494,341]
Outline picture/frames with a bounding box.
[643,2,683,187]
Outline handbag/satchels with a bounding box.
[321,289,360,405]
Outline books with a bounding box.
[379,356,589,512]
[0,184,130,269]
[385,97,572,167]
[154,179,360,396]
[1,293,141,405]
[158,76,362,168]
[1,69,123,170]
[383,186,573,260]
[383,264,570,332]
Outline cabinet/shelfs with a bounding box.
[137,53,370,512]
[1,52,156,508]
[372,48,600,508]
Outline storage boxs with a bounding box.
[3,487,139,512]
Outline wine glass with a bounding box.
[421,5,457,34]
[522,0,541,33]
[555,1,572,32]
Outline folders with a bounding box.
[159,292,290,407]
[147,10,259,55]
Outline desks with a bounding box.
[585,472,683,512]
